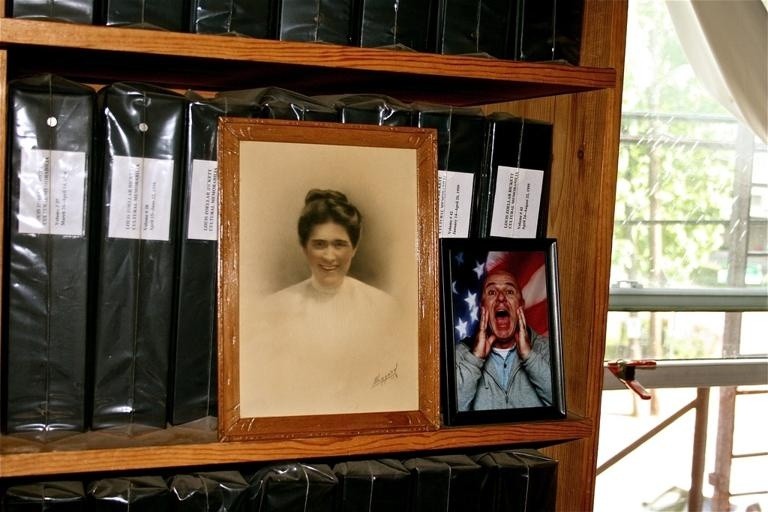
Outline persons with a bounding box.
[451,264,554,411]
[257,183,402,348]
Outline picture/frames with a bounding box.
[215,115,439,442]
[441,238,564,426]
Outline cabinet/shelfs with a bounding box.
[0,0,631,511]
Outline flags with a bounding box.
[449,248,552,342]
